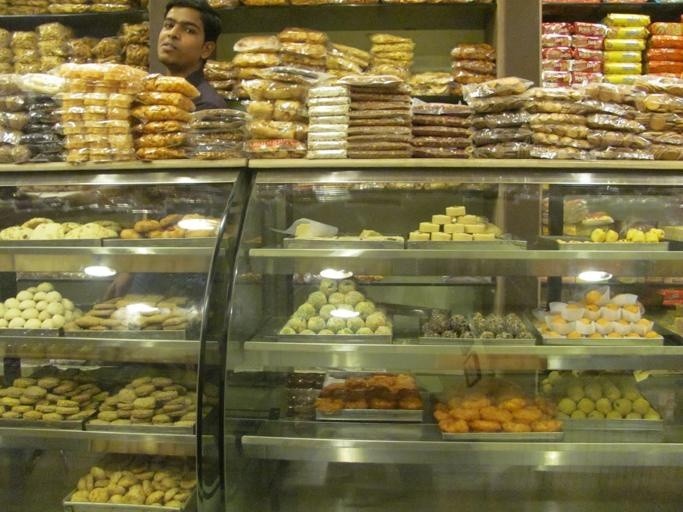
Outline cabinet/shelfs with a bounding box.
[0,155,248,512]
[219,158,683,512]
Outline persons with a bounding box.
[102,0,228,303]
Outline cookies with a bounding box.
[0,0,683,512]
